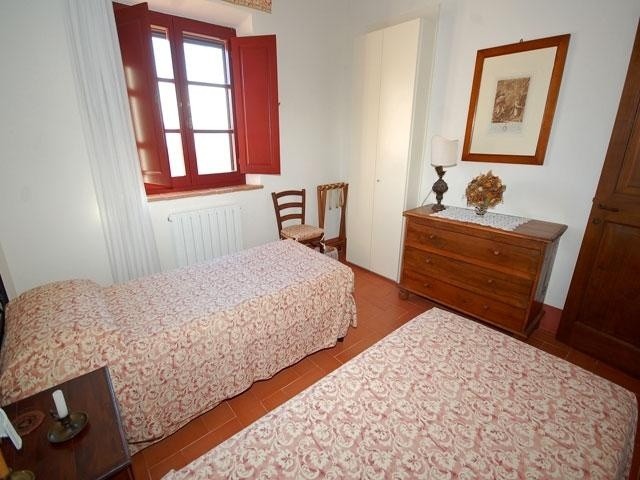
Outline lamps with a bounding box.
[431,135,459,211]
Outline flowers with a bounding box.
[466,169,507,208]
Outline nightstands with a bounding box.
[0,365,136,479]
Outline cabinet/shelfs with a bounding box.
[399,203,570,342]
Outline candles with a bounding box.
[52,390,68,419]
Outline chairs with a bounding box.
[271,189,325,254]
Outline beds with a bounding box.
[2,239,356,458]
[159,308,637,479]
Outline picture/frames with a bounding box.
[462,34,570,165]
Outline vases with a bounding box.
[475,205,488,216]
